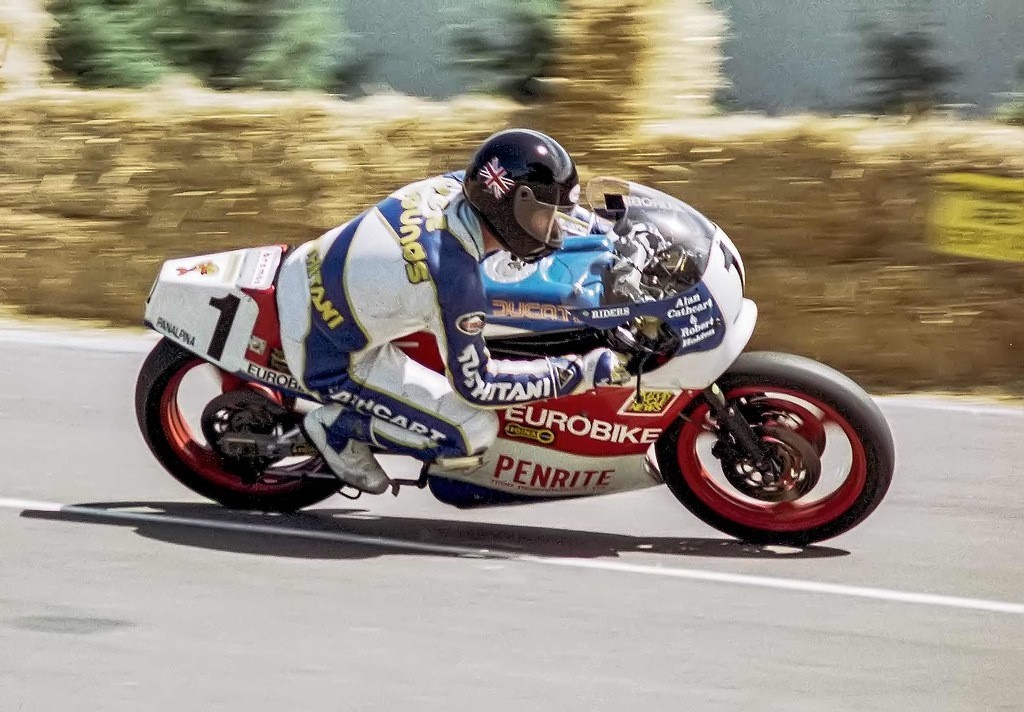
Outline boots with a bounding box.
[303,402,390,495]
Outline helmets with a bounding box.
[461,128,581,265]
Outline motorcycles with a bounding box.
[135,175,894,546]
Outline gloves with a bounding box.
[582,347,631,391]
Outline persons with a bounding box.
[274,128,619,494]
